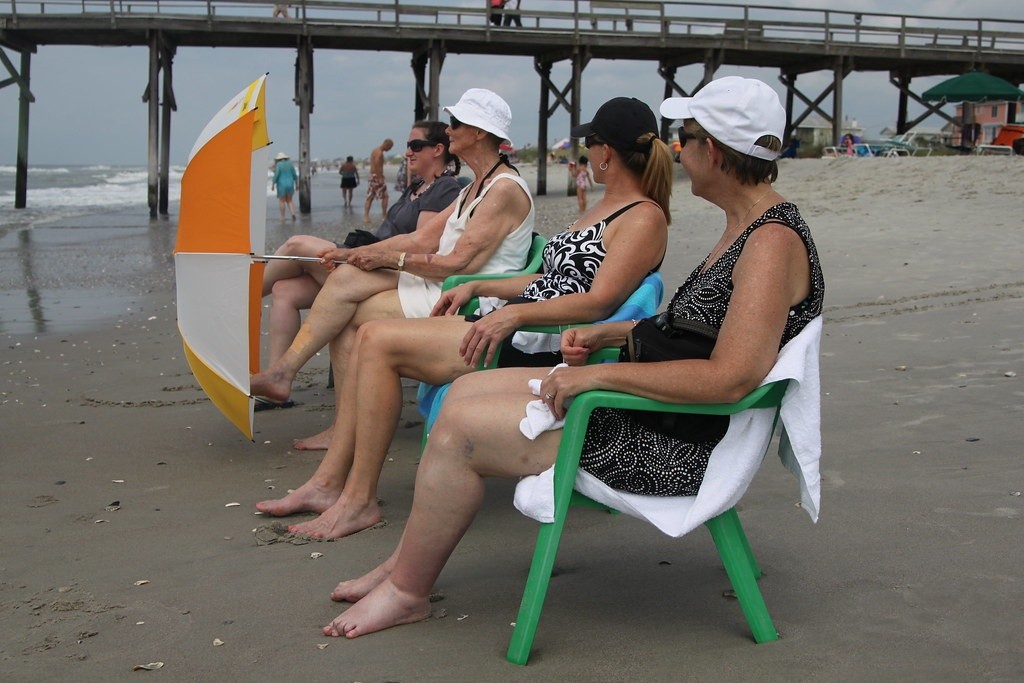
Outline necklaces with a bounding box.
[701,189,773,277]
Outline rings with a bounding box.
[545,392,555,400]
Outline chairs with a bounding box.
[327,235,823,665]
[823,126,1024,157]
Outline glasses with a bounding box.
[584,135,610,149]
[407,139,437,152]
[449,115,463,129]
[677,125,707,148]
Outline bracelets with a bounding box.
[632,318,637,326]
[398,251,406,271]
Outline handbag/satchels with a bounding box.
[343,229,381,248]
[618,313,729,444]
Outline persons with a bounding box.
[571,155,593,213]
[273,2,288,18]
[255,121,463,412]
[339,155,359,207]
[321,75,826,639]
[272,151,299,225]
[363,138,394,223]
[490,0,522,27]
[256,97,675,539]
[250,88,537,450]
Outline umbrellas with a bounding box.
[176,71,402,444]
[921,71,1024,147]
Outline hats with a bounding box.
[276,152,290,160]
[443,87,512,147]
[660,75,786,161]
[570,97,660,156]
